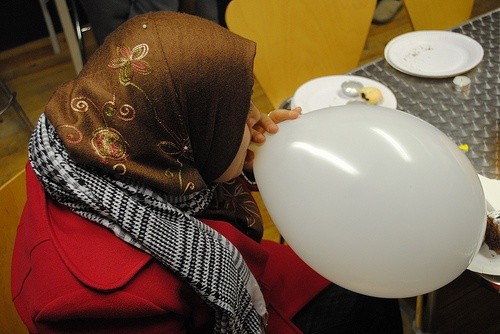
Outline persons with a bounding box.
[10,10,404,334]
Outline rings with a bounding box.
[267,111,271,117]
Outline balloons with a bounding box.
[248,101,486,298]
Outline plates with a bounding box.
[384,31,485,78]
[454,179,500,275]
[290,75,397,125]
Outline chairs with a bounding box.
[224,0,475,111]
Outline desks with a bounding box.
[279,5,500,291]
